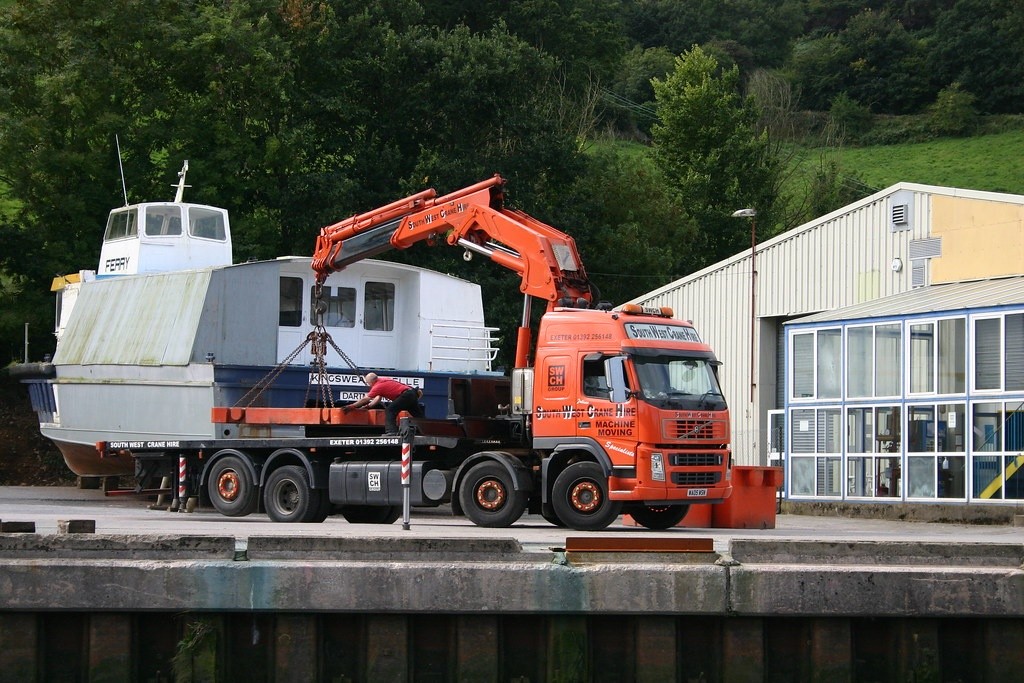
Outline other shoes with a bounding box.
[386,433,399,436]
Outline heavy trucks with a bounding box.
[187,169,733,530]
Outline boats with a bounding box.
[28,133,232,479]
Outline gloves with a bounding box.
[341,404,356,414]
[361,404,370,409]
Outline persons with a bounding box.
[341,372,426,436]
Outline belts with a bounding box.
[403,388,413,393]
